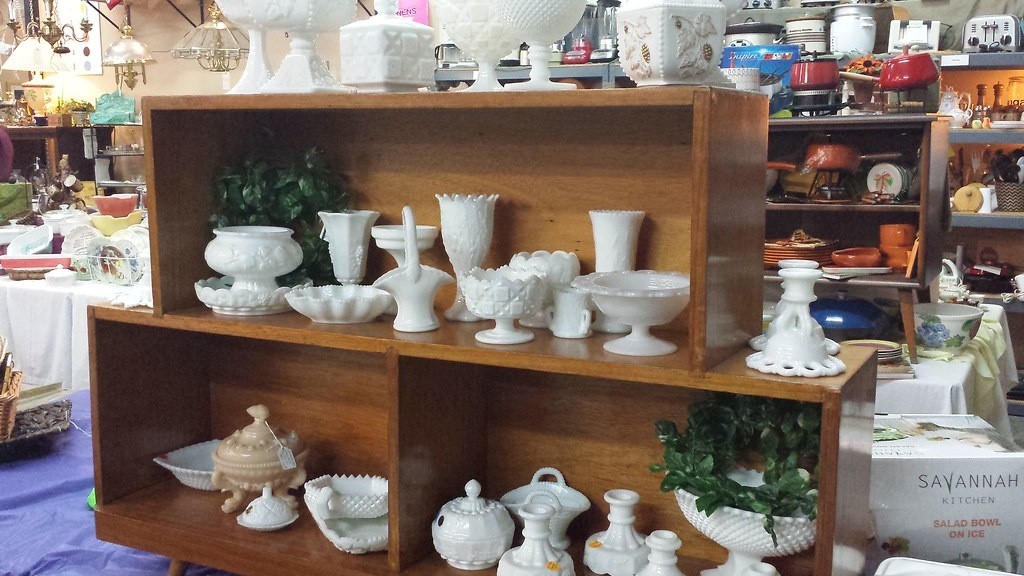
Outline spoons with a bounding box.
[982,144,1024,183]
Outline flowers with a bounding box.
[843,51,896,75]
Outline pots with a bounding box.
[791,45,939,175]
[808,290,984,351]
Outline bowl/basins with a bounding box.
[831,224,916,270]
[0,194,144,287]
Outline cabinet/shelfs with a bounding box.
[85,90,879,576]
[751,113,954,365]
[89,124,146,196]
[823,52,1024,399]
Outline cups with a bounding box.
[63,175,84,193]
[975,75,1024,120]
[546,210,648,338]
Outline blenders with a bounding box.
[563,0,622,64]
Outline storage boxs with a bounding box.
[720,44,805,119]
[867,412,1023,576]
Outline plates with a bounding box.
[152,436,308,491]
[764,162,920,363]
[988,121,1024,129]
[61,212,152,286]
[193,275,393,326]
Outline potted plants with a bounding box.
[72,106,87,124]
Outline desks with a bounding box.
[0,122,115,186]
[875,304,1019,445]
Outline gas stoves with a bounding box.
[790,91,851,117]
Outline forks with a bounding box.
[970,152,982,174]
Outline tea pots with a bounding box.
[935,85,973,130]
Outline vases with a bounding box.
[851,81,874,107]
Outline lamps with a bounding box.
[101,0,157,90]
[170,2,250,72]
[0,0,93,54]
[2,37,70,116]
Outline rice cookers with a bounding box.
[724,0,878,56]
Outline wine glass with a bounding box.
[370,191,580,329]
